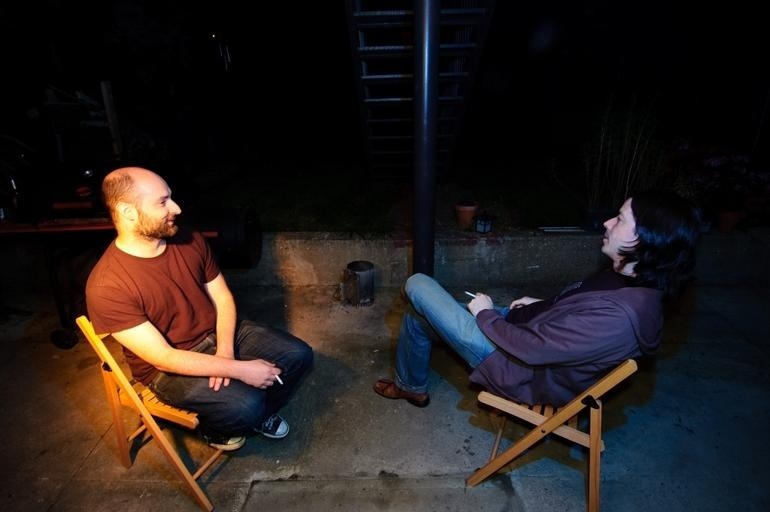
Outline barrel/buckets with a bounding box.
[347,259,374,307]
[454,205,478,229]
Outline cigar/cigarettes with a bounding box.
[273,374,286,385]
[463,289,477,299]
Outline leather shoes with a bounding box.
[374,380,429,407]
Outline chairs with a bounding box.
[73,314,224,512]
[464,359,639,512]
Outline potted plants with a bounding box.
[573,82,662,231]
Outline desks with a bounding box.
[1,214,112,239]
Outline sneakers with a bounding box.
[204,413,290,450]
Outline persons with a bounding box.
[373,190,708,409]
[85,167,314,452]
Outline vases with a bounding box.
[455,199,480,231]
[717,209,746,233]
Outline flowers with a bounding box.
[695,151,770,209]
